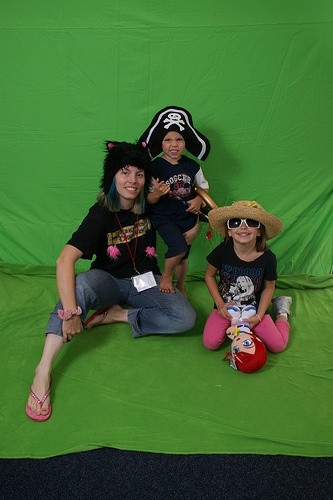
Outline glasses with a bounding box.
[226,217,260,229]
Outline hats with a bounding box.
[99,140,148,194]
[137,106,211,161]
[208,201,282,241]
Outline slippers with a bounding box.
[82,306,108,329]
[25,384,52,421]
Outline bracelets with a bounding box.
[57,306,83,320]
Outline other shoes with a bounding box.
[271,295,292,314]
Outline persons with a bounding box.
[136,106,209,300]
[203,200,293,353]
[226,305,267,373]
[25,141,197,421]
